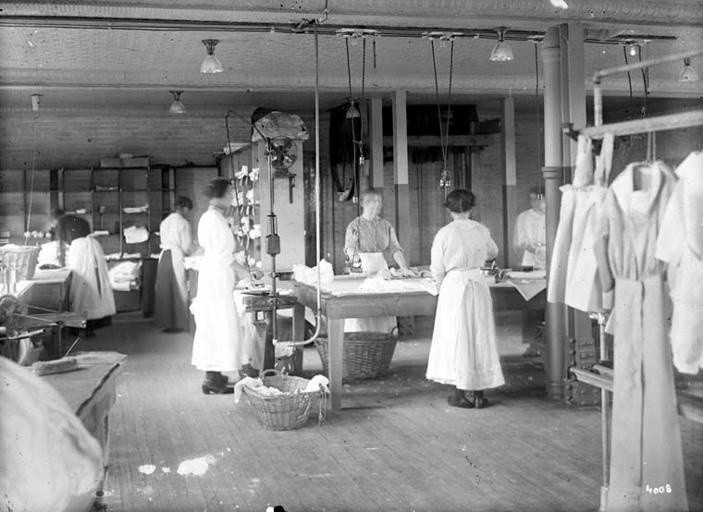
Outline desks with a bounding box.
[293,267,547,411]
[19,359,120,512]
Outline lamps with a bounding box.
[677,56,700,84]
[346,96,361,119]
[199,39,224,75]
[167,89,187,115]
[488,26,512,63]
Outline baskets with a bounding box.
[308,327,402,382]
[242,369,329,431]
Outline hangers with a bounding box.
[631,118,660,170]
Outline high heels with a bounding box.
[202,380,234,394]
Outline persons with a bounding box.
[154,196,199,333]
[512,186,546,356]
[46,208,116,339]
[343,188,416,336]
[191,179,259,394]
[424,189,507,409]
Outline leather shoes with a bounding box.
[447,396,473,408]
[473,398,487,409]
